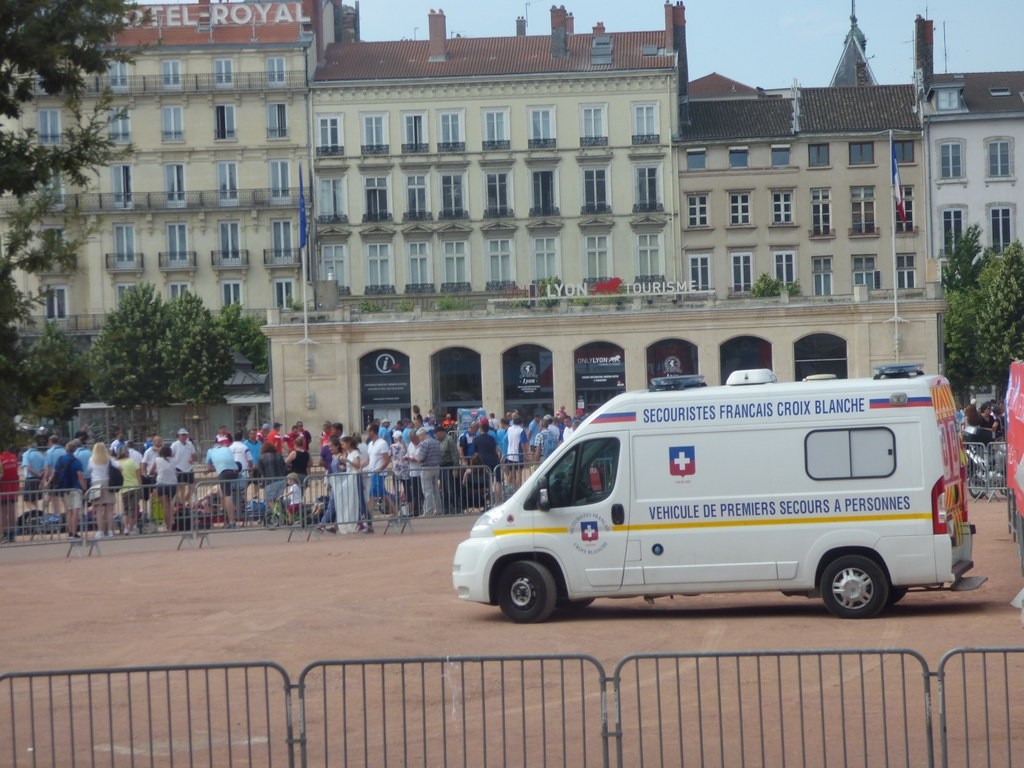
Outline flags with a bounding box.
[892,137,907,223]
[299,162,306,249]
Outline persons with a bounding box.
[959,398,1004,481]
[0,405,592,544]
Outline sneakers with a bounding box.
[225,522,235,529]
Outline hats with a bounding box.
[433,426,443,433]
[381,419,391,424]
[273,423,283,428]
[263,424,271,430]
[392,431,403,438]
[177,428,188,434]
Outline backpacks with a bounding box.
[108,461,124,492]
[49,457,76,495]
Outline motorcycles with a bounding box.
[966,443,1008,499]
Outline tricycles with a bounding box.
[264,495,312,531]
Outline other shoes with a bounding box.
[1,532,16,544]
[356,524,364,531]
[94,532,104,539]
[69,532,81,540]
[363,529,373,533]
[314,525,325,533]
[107,531,114,538]
[124,527,139,535]
[326,527,337,533]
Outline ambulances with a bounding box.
[451,362,975,624]
